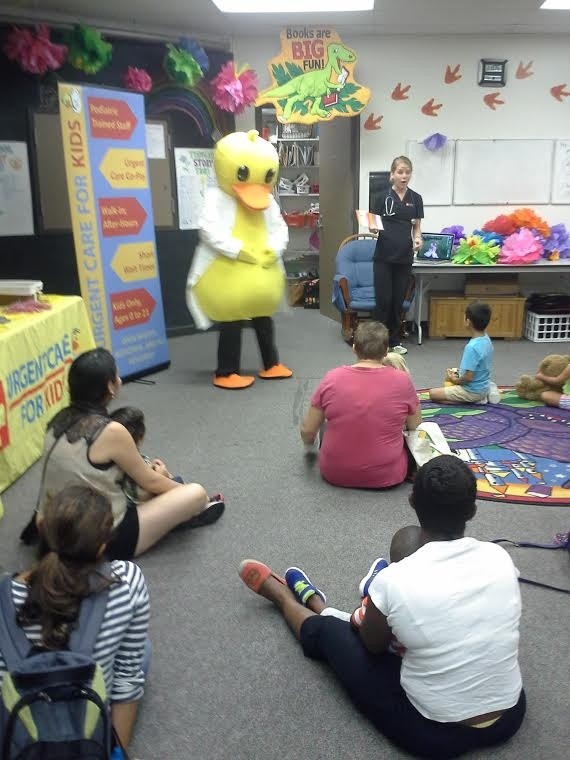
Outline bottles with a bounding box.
[489,382,500,404]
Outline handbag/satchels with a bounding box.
[528,294,570,314]
[20,510,41,546]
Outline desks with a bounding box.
[0,294,96,494]
[411,258,570,345]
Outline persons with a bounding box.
[111,406,224,504]
[19,347,225,561]
[429,302,501,405]
[187,129,292,389]
[300,321,420,490]
[368,155,424,354]
[285,523,429,683]
[535,364,570,410]
[382,351,408,372]
[236,455,527,760]
[0,486,150,760]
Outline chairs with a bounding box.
[332,233,416,337]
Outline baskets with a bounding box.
[524,311,570,342]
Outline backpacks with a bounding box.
[0,561,130,760]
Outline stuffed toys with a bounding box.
[516,354,570,401]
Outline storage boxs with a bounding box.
[427,274,527,341]
[524,310,570,343]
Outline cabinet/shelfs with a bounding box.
[255,103,320,254]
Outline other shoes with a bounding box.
[172,476,184,484]
[351,596,368,627]
[394,346,407,355]
[238,560,285,599]
[177,501,226,528]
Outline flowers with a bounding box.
[441,208,570,263]
[4,16,260,116]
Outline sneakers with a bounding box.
[285,567,326,609]
[358,557,388,599]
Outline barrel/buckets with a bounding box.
[287,272,308,288]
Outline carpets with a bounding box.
[407,386,570,506]
